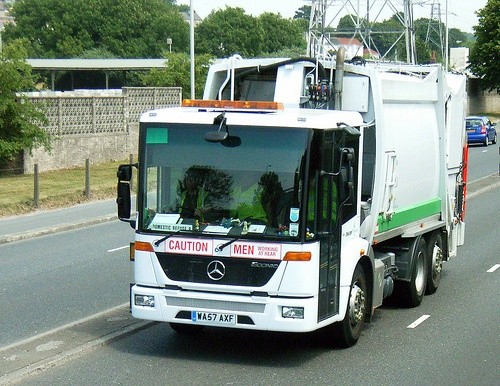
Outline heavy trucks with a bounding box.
[116,39,468,348]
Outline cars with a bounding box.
[466,115,498,148]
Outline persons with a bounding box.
[301,157,337,231]
[174,172,215,222]
[225,168,272,226]
